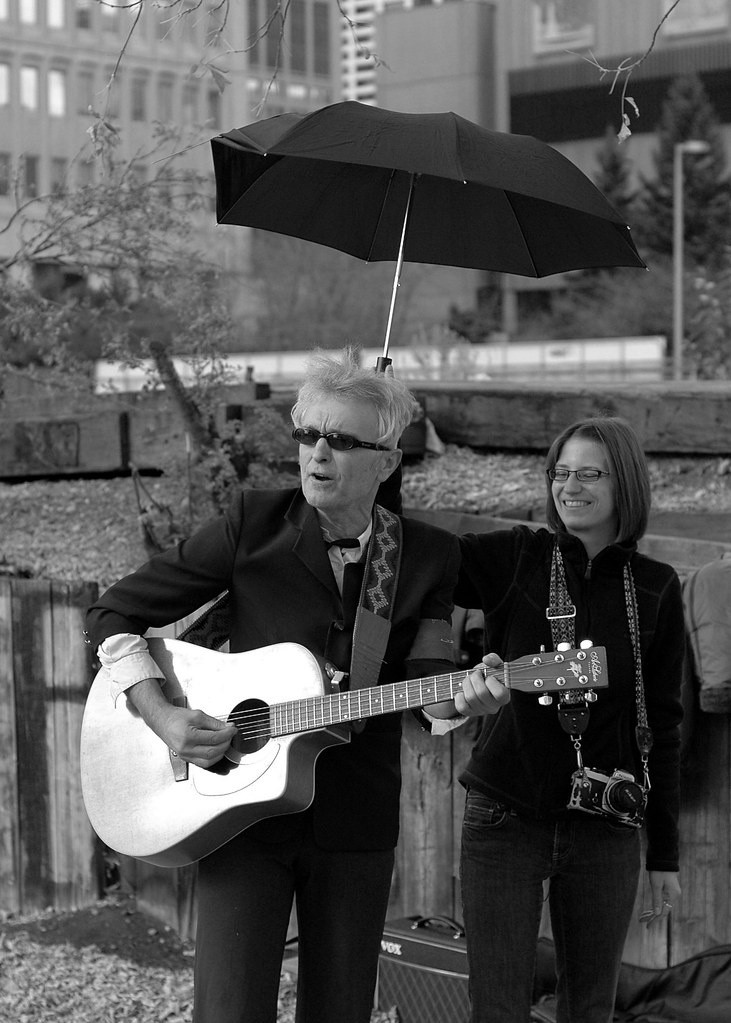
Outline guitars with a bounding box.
[79,637,610,867]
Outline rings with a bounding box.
[663,901,673,908]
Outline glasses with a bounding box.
[292,427,391,452]
[546,467,610,482]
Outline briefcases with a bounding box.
[527,991,558,1023]
[377,915,476,1023]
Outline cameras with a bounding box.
[567,768,648,829]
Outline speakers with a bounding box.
[374,917,471,1023]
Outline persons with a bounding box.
[84,342,510,1023]
[379,364,687,1023]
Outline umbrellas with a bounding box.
[211,102,651,381]
